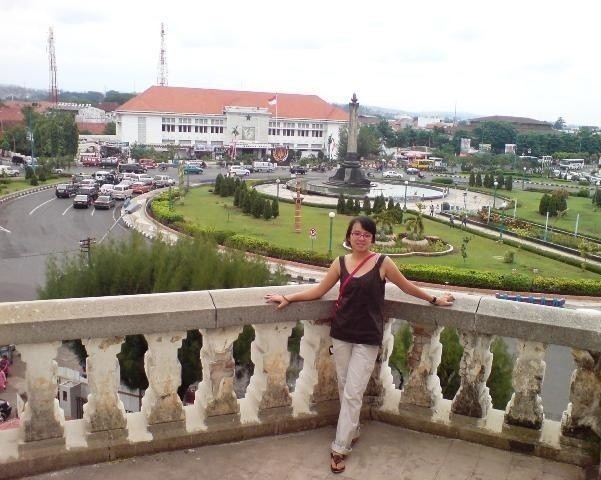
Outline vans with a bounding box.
[253,161,275,173]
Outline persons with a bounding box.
[430,204,468,229]
[261,215,455,475]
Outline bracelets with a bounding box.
[429,296,438,305]
[282,294,294,304]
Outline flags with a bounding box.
[267,95,276,105]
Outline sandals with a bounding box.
[330,453,345,473]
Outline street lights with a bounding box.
[493,181,499,210]
[522,167,527,189]
[275,179,281,199]
[327,211,336,258]
[403,181,409,209]
[500,202,507,242]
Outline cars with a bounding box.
[407,168,420,175]
[534,167,601,186]
[384,170,403,179]
[0,152,177,210]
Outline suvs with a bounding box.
[184,165,203,174]
[230,166,250,176]
[290,165,308,175]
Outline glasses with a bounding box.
[351,232,373,240]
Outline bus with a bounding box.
[411,159,435,171]
[559,159,584,170]
[538,156,553,164]
